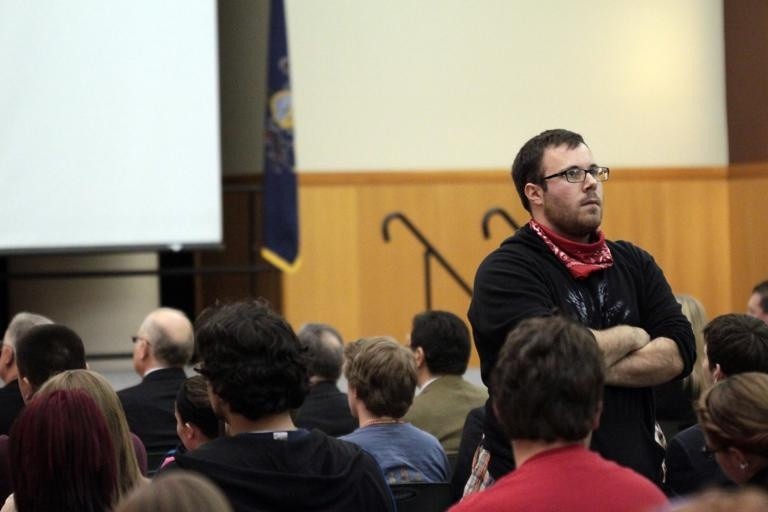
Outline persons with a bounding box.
[693,369,767,488]
[656,295,714,442]
[445,315,673,511]
[0,300,491,511]
[665,311,768,495]
[747,279,767,321]
[466,128,697,481]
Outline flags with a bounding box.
[260,0,304,274]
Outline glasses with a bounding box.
[534,167,610,183]
[699,442,734,467]
